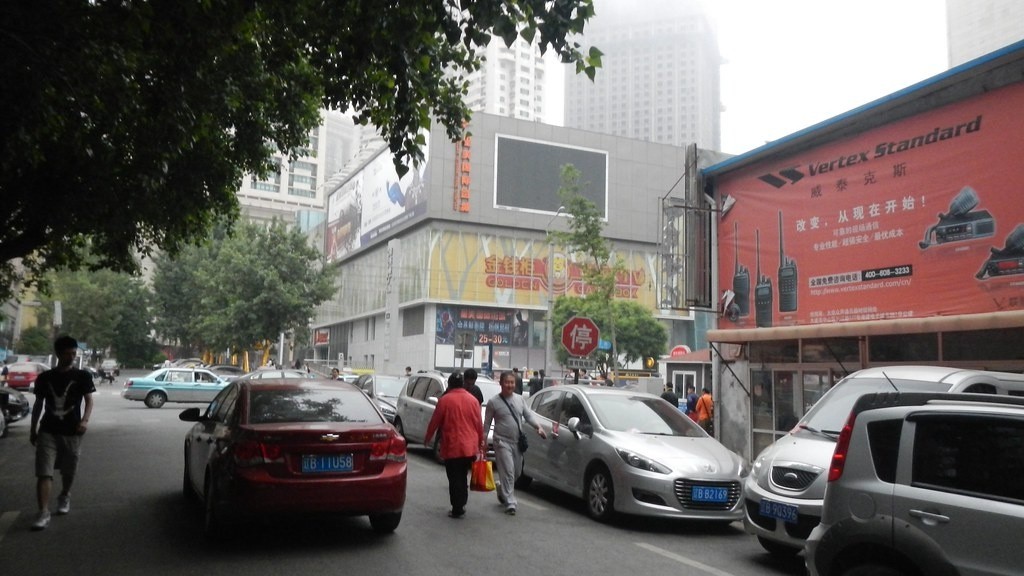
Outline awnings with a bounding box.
[708,309,1024,397]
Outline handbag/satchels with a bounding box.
[470,450,496,492]
[518,432,528,452]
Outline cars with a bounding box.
[5,363,44,390]
[514,385,750,530]
[392,369,527,466]
[351,373,409,424]
[120,365,231,409]
[0,385,29,440]
[153,357,360,383]
[179,378,408,548]
[99,359,120,377]
[522,378,531,399]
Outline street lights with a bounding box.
[544,204,567,377]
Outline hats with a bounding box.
[448,373,462,389]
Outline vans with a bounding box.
[801,390,1024,576]
[742,365,1024,560]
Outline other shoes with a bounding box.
[33,512,51,528]
[58,491,72,513]
[506,508,515,515]
[495,480,506,504]
[448,505,465,518]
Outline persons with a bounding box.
[406,367,411,375]
[28,335,96,528]
[0,359,9,387]
[425,372,484,517]
[301,362,311,373]
[270,358,276,364]
[527,369,545,397]
[112,367,121,376]
[98,365,117,385]
[483,371,548,515]
[464,368,485,406]
[329,367,345,381]
[580,369,592,385]
[512,367,523,395]
[660,382,678,408]
[291,358,301,368]
[685,385,713,430]
